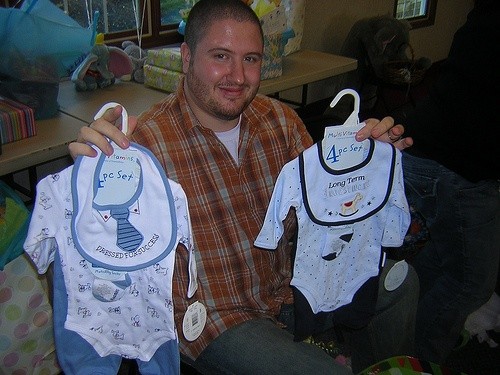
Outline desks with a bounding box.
[0,47,359,207]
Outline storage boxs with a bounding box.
[144,48,184,92]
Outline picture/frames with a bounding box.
[393,0,437,29]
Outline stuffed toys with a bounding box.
[120,41,148,82]
[71,33,132,91]
[331,16,410,110]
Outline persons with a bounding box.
[67,0,420,375]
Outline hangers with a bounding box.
[281,89,402,173]
[35,101,188,203]
